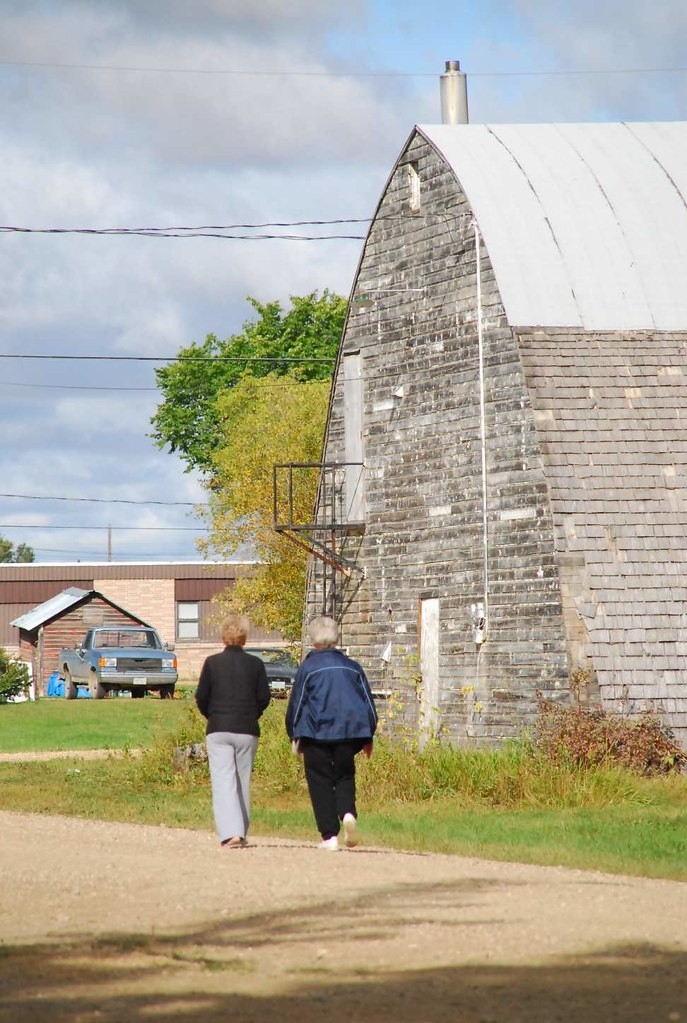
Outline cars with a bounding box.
[243,647,301,699]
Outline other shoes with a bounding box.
[225,837,242,848]
[343,812,358,848]
[316,836,338,851]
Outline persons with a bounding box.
[195,615,272,848]
[285,617,378,851]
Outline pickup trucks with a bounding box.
[59,625,178,700]
